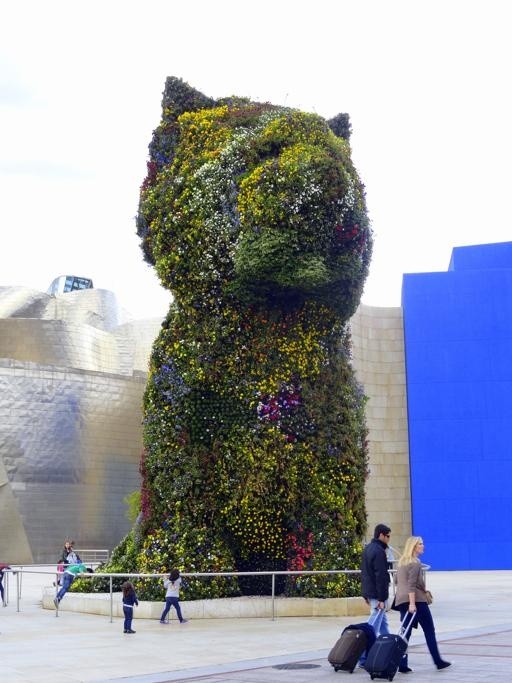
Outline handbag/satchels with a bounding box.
[424,591,433,604]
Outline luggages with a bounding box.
[327,607,416,681]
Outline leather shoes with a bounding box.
[124,630,136,633]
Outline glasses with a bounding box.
[385,535,389,538]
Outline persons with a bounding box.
[53,564,93,608]
[393,536,451,672]
[160,568,188,624]
[359,524,391,669]
[122,582,138,633]
[0,563,17,607]
[53,541,82,587]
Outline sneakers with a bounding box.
[436,661,453,671]
